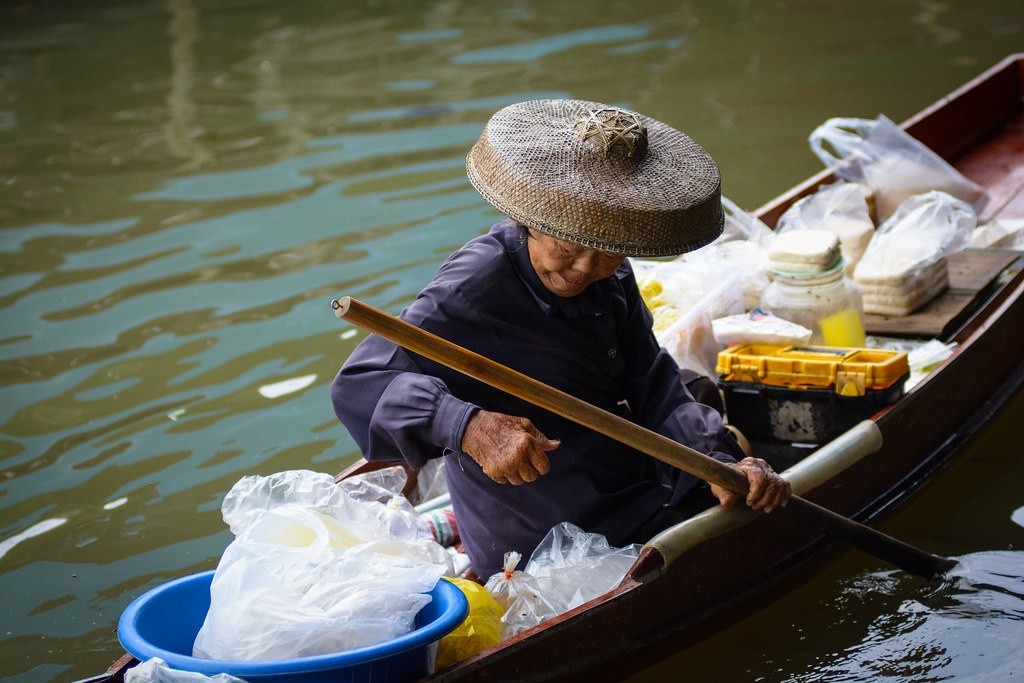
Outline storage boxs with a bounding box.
[717,343,911,449]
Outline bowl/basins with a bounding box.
[116,563,471,683]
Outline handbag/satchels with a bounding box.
[806,113,991,228]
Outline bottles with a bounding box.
[762,262,870,352]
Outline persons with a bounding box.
[330,98,792,582]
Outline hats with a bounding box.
[464,99,725,256]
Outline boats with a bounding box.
[72,51,1024,682]
